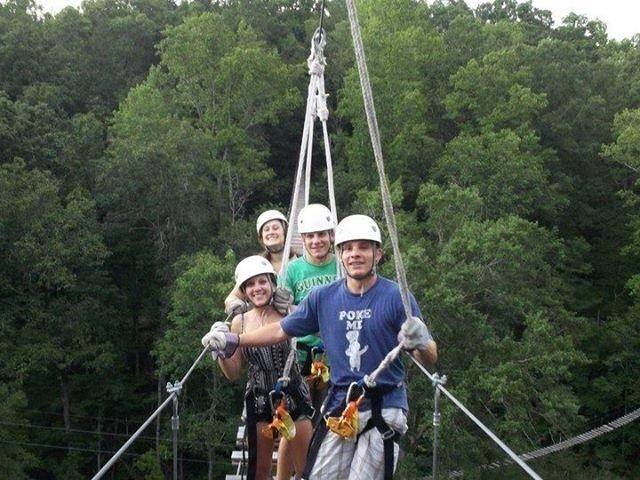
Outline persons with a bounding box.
[225,210,303,445]
[210,256,321,479]
[201,213,440,479]
[272,202,346,480]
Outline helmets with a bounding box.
[256,210,289,236]
[297,204,334,234]
[235,255,276,291]
[334,214,382,250]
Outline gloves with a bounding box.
[397,316,429,351]
[199,321,240,361]
[224,298,247,315]
[272,286,292,315]
